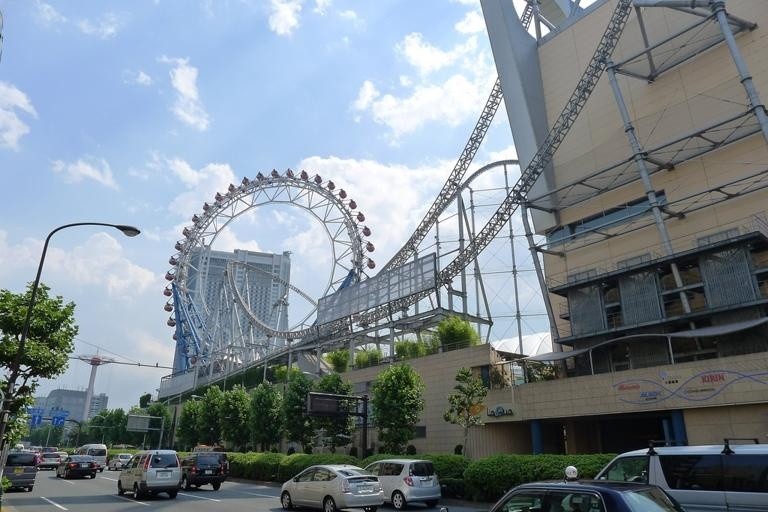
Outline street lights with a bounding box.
[0,222,140,455]
[94,415,106,444]
[191,394,205,400]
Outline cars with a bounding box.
[55,455,96,480]
[177,455,223,492]
[484,465,685,512]
[106,453,134,471]
[13,444,69,469]
[279,463,384,512]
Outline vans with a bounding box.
[592,437,768,512]
[0,451,38,493]
[361,458,441,512]
[76,444,107,472]
[116,449,183,500]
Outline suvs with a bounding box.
[191,451,230,484]
